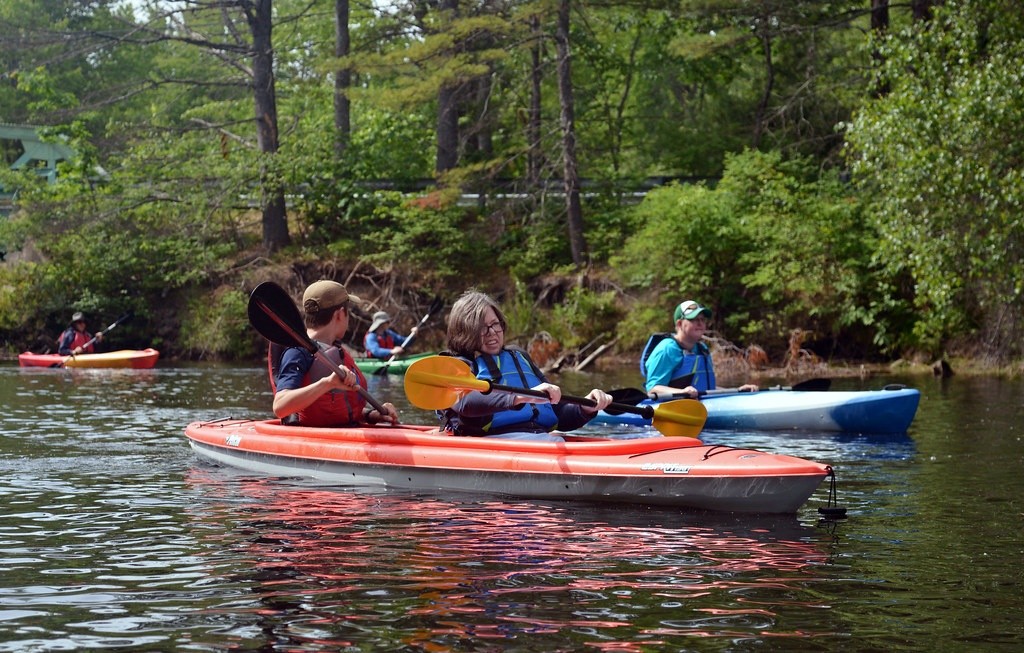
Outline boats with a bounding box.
[586,384,921,435]
[18,347,160,370]
[180,413,851,524]
[351,349,441,374]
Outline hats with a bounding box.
[72,312,84,321]
[302,279,349,313]
[673,300,712,327]
[368,312,390,333]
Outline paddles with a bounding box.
[374,297,447,376]
[404,354,708,442]
[603,376,833,416]
[48,314,134,370]
[245,278,395,420]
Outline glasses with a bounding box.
[687,318,708,323]
[480,322,504,336]
[678,304,705,319]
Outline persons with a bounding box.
[437,288,614,445]
[363,312,418,360]
[58,312,103,356]
[273,280,398,427]
[645,300,760,399]
